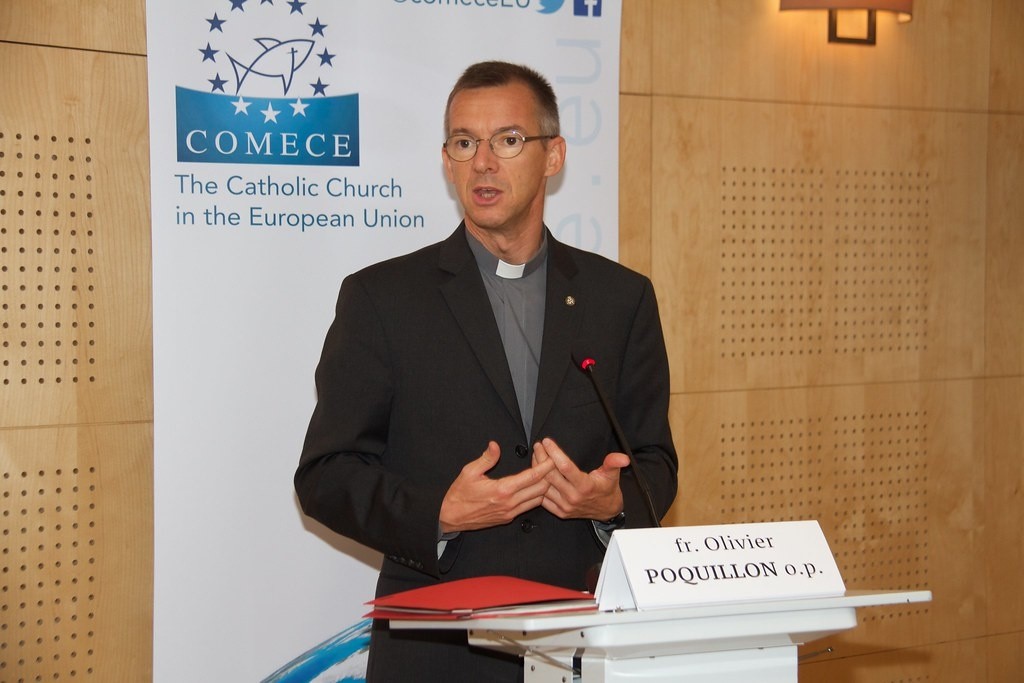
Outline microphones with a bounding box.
[570,339,662,528]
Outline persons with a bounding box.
[295,60,677,683]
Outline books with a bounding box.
[361,570,601,625]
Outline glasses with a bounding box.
[440,131,557,163]
